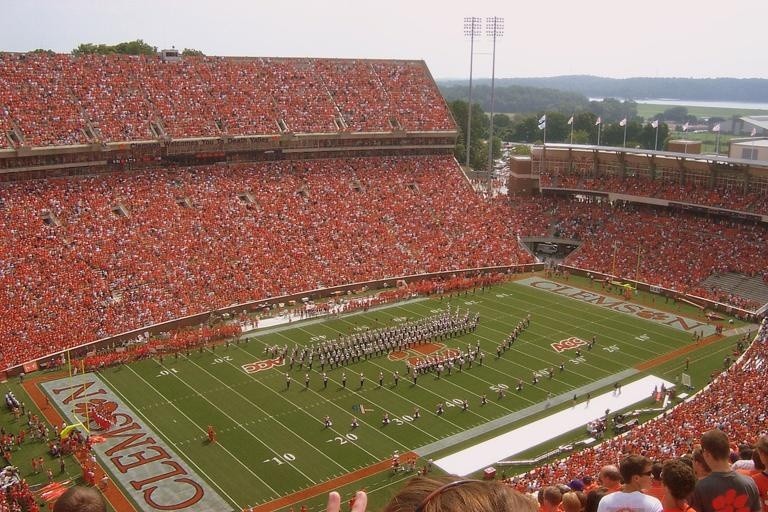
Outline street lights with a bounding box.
[464,16,481,170]
[487,16,504,191]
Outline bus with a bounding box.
[536,242,559,255]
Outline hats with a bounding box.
[565,480,585,490]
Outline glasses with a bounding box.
[636,471,652,476]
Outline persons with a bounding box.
[0,48,768,512]
